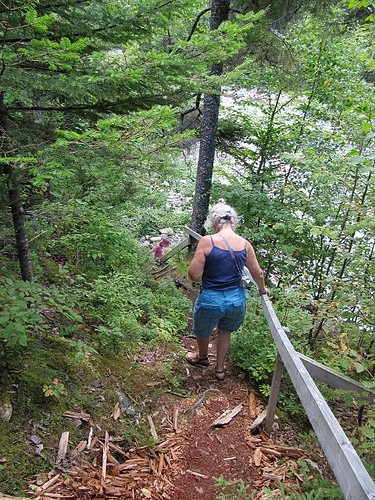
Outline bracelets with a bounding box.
[258,288,265,292]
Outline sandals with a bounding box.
[186,352,210,368]
[215,367,226,382]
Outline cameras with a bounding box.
[239,275,251,289]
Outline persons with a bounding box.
[185,202,271,381]
[150,237,170,260]
